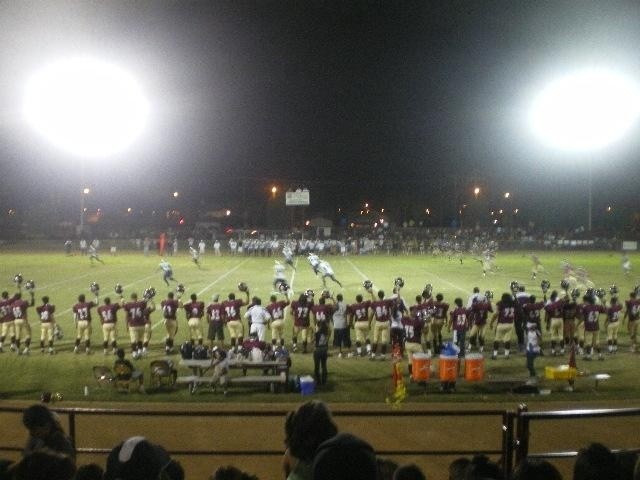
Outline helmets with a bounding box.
[422,284,432,298]
[542,279,569,289]
[91,282,99,292]
[14,276,34,289]
[279,283,288,292]
[238,282,247,291]
[144,288,156,299]
[395,278,404,287]
[511,280,518,292]
[485,290,494,298]
[363,280,372,289]
[571,285,618,297]
[115,285,122,293]
[177,285,185,292]
[305,290,330,298]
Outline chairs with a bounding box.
[92,363,134,397]
[149,360,177,394]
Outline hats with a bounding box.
[313,433,376,479]
[107,436,169,480]
[213,294,220,300]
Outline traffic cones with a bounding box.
[84,386,89,397]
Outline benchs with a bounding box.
[176,355,288,396]
[405,370,611,396]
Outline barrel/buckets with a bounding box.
[465,353,483,382]
[438,354,458,384]
[411,352,431,384]
[299,376,317,398]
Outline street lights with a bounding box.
[78,187,91,232]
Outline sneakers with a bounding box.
[74,349,117,355]
[552,349,565,355]
[574,348,605,361]
[608,347,640,354]
[526,376,536,385]
[293,346,307,353]
[338,350,386,360]
[133,350,146,359]
[0,347,57,355]
[466,348,510,360]
[165,348,175,353]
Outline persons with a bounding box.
[189,245,201,268]
[530,253,549,281]
[273,259,286,280]
[481,245,496,277]
[160,259,178,285]
[62,223,586,264]
[317,262,342,289]
[2,405,640,480]
[282,247,296,271]
[0,276,639,396]
[307,251,320,274]
[89,241,104,267]
[621,251,632,276]
